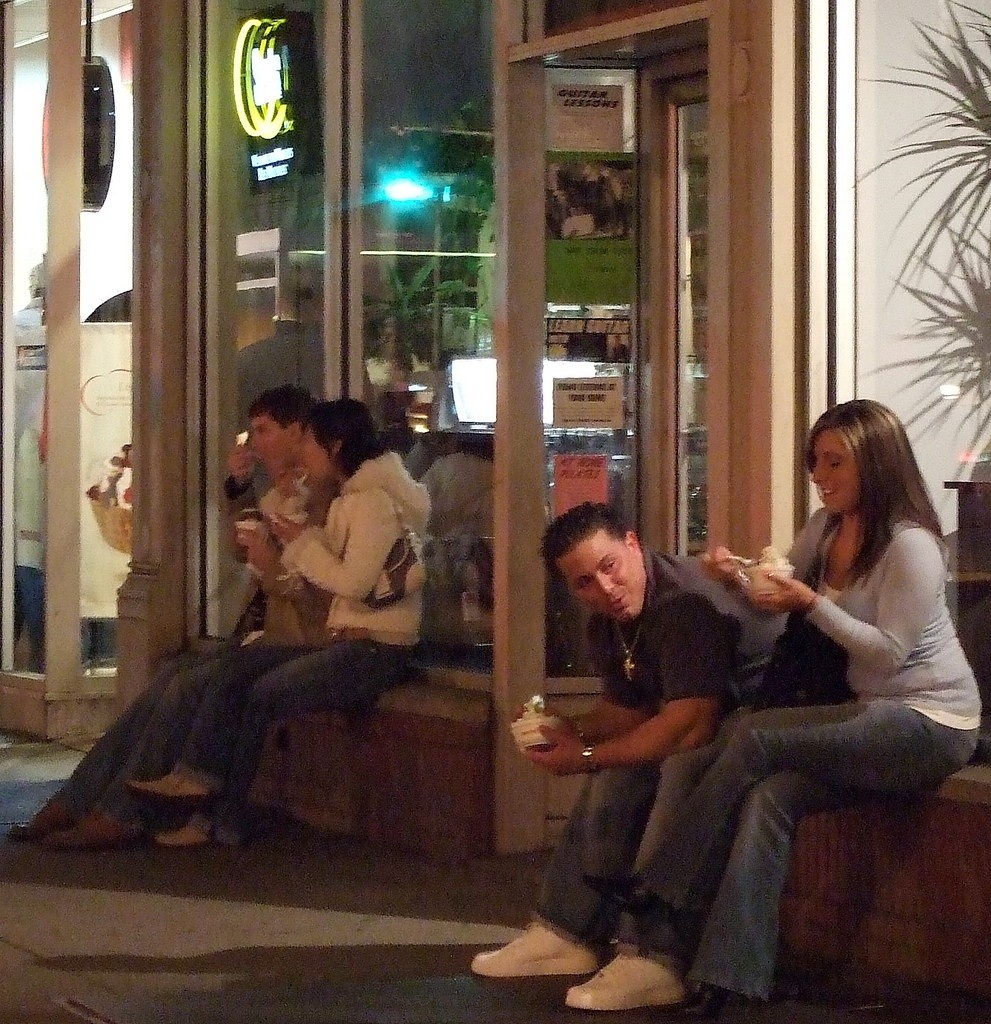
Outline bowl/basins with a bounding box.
[271,510,309,529]
[511,716,561,752]
[235,520,261,541]
[745,566,795,595]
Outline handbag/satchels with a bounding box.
[338,491,427,609]
[750,511,856,712]
[449,538,495,609]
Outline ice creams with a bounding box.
[745,545,795,595]
[511,695,565,747]
[83,443,133,554]
[234,507,265,539]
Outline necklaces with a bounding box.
[614,618,639,681]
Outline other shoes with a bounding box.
[42,807,142,851]
[6,794,82,842]
[153,812,243,846]
[124,770,209,803]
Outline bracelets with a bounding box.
[581,746,594,774]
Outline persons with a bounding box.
[469,498,787,979]
[128,398,430,848]
[564,397,985,1010]
[13,382,339,848]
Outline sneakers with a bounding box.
[472,920,598,976]
[564,950,685,1009]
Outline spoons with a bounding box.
[726,556,759,566]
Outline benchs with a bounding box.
[245,685,493,852]
[785,763,991,1024]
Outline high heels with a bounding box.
[586,873,685,925]
[647,980,756,1024]
